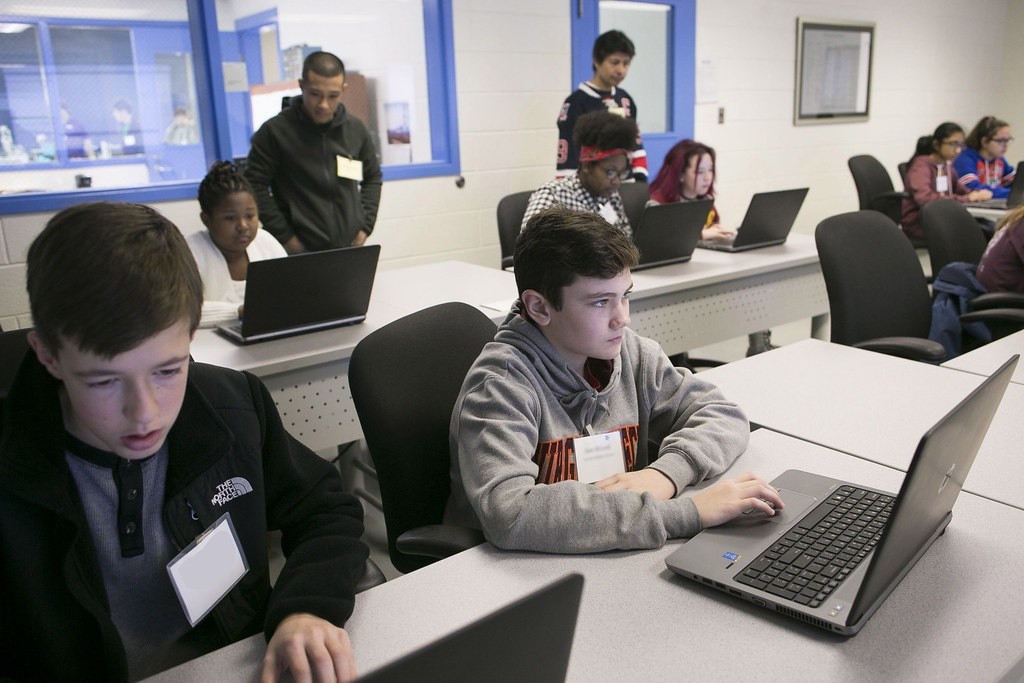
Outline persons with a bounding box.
[644,139,775,358]
[976,203,1024,339]
[0,201,370,683]
[899,122,992,241]
[519,109,636,247]
[555,30,649,184]
[953,116,1015,198]
[61,107,95,158]
[181,161,292,328]
[440,206,783,554]
[164,110,198,145]
[111,100,142,147]
[244,51,383,255]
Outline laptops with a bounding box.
[963,161,1024,210]
[696,188,810,253]
[660,353,1021,637]
[213,244,381,344]
[630,198,715,274]
[347,572,586,683]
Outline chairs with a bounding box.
[618,182,650,235]
[346,302,500,574]
[920,199,1024,310]
[848,156,910,226]
[497,191,536,270]
[813,208,1024,365]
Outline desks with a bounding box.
[137,234,1024,683]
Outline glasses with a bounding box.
[940,139,968,152]
[596,163,630,180]
[988,136,1015,146]
